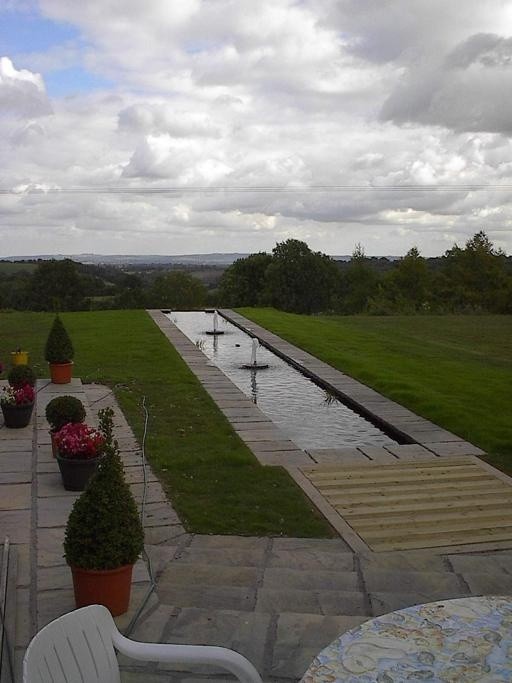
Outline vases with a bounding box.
[55,455,99,493]
[4,407,33,432]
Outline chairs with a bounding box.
[23,604,266,683]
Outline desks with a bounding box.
[295,594,512,681]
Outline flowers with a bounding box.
[4,385,39,404]
[54,426,105,456]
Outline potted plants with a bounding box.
[45,395,86,457]
[44,320,76,383]
[59,455,135,626]
